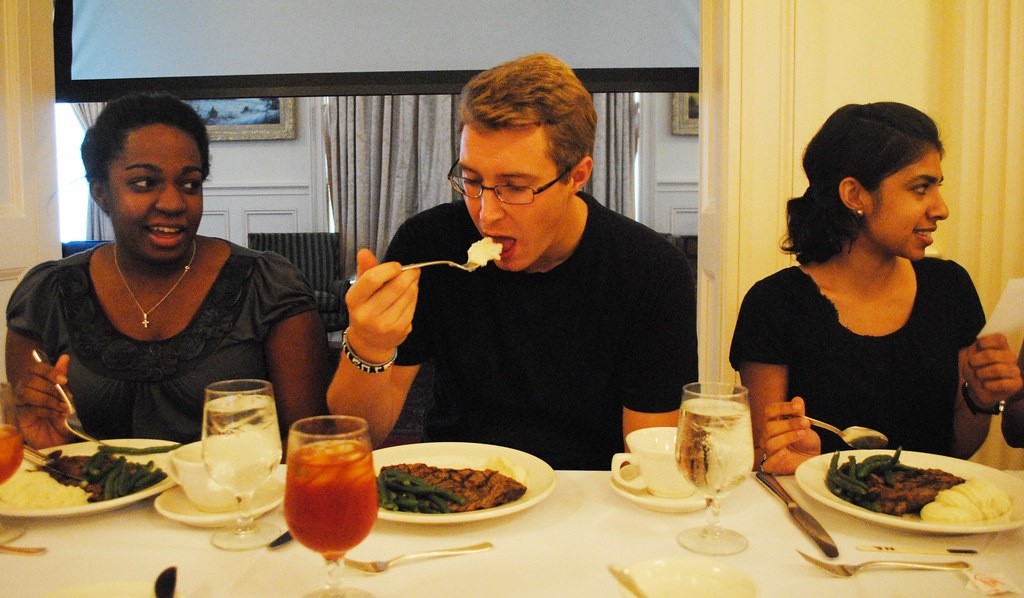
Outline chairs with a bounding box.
[247,232,349,337]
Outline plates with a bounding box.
[372,440,557,524]
[795,449,1023,534]
[153,477,284,528]
[609,464,707,514]
[0,438,183,518]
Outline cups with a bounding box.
[607,554,760,598]
[162,441,241,512]
[611,427,698,498]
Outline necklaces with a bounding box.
[114,239,194,327]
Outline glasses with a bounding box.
[446,158,570,205]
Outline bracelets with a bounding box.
[340,326,399,372]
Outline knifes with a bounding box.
[266,530,293,548]
[23,444,85,482]
[756,470,839,558]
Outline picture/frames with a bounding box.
[671,92,699,136]
[181,95,296,140]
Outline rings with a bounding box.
[760,454,769,464]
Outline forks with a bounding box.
[795,548,970,578]
[349,262,481,286]
[33,348,105,445]
[338,542,495,575]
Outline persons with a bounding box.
[728,102,1024,474]
[0,92,327,450]
[324,56,694,471]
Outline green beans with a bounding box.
[828,445,917,515]
[374,469,467,515]
[79,444,181,500]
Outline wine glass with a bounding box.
[283,415,374,598]
[0,381,25,546]
[201,378,282,552]
[676,381,755,557]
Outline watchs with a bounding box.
[961,382,1005,416]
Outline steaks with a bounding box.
[377,463,527,513]
[44,455,118,494]
[870,467,966,516]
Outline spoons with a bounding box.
[783,412,889,449]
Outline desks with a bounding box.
[0,466,1024,598]
